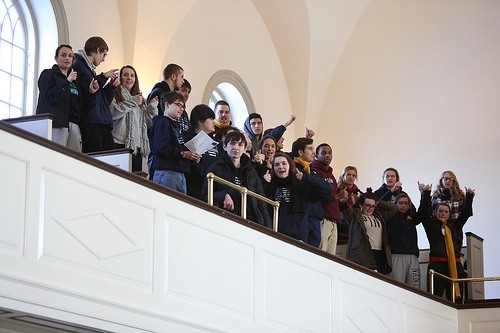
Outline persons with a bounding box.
[431,171,466,303]
[148,63,340,256]
[371,168,415,278]
[71,37,121,157]
[416,180,475,303]
[378,180,432,292]
[201,129,272,233]
[342,192,399,278]
[337,166,365,263]
[262,151,333,253]
[37,43,84,153]
[112,63,159,181]
[148,91,203,195]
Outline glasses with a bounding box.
[397,201,409,206]
[442,177,454,182]
[362,203,375,210]
[100,49,107,58]
[173,102,186,110]
